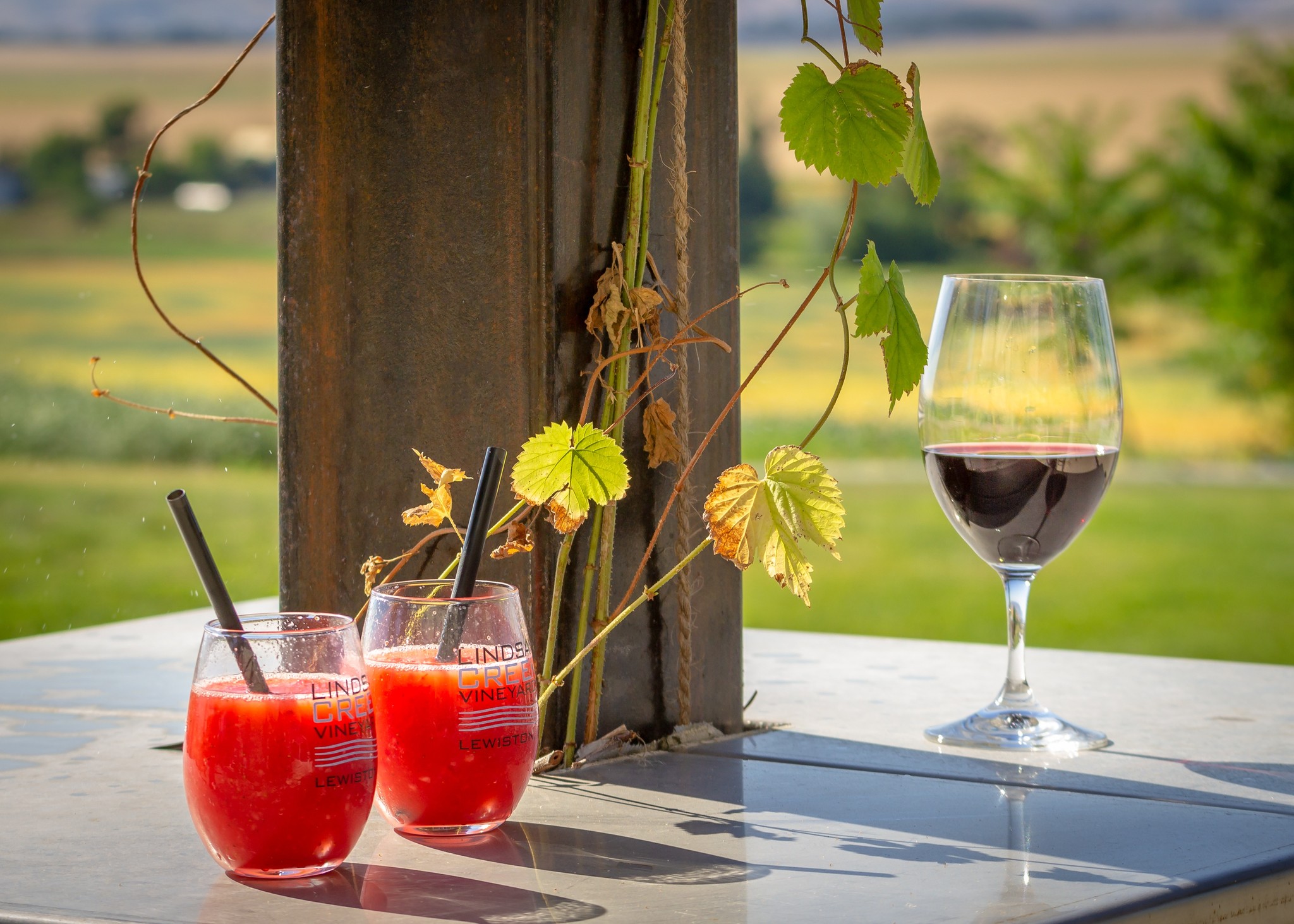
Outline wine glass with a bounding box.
[917,269,1123,753]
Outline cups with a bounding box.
[182,610,377,878]
[361,578,539,836]
